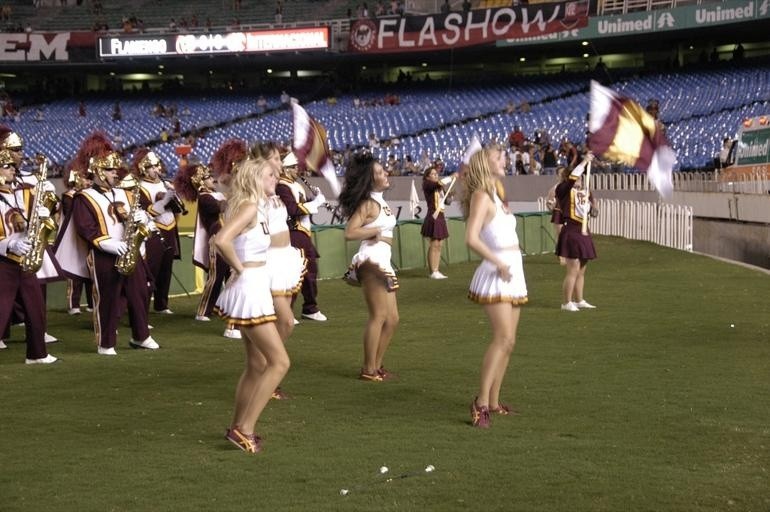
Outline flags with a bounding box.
[589,81,676,203]
[291,99,342,199]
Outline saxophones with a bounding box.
[114,174,152,276]
[14,169,57,274]
[21,153,62,246]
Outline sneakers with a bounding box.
[98,346,118,356]
[68,308,81,315]
[195,315,211,322]
[129,335,160,350]
[154,308,172,314]
[469,396,490,427]
[430,271,448,279]
[361,365,399,383]
[489,400,514,416]
[223,329,242,339]
[561,300,596,311]
[270,389,295,400]
[44,332,58,343]
[225,426,262,452]
[302,311,327,321]
[25,354,58,365]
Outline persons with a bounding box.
[352,94,401,107]
[647,99,660,119]
[331,132,447,176]
[709,48,721,64]
[3,125,328,365]
[545,151,598,311]
[719,138,734,169]
[2,1,403,34]
[466,144,529,426]
[733,43,745,60]
[507,98,532,114]
[423,168,461,280]
[1,72,292,145]
[397,69,433,82]
[505,125,612,176]
[213,159,291,452]
[337,153,402,381]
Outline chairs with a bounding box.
[1,1,384,34]
[1,69,770,184]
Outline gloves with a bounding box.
[135,210,148,224]
[8,237,33,256]
[162,189,175,206]
[117,241,128,256]
[38,206,50,218]
[311,185,326,207]
[43,181,55,192]
